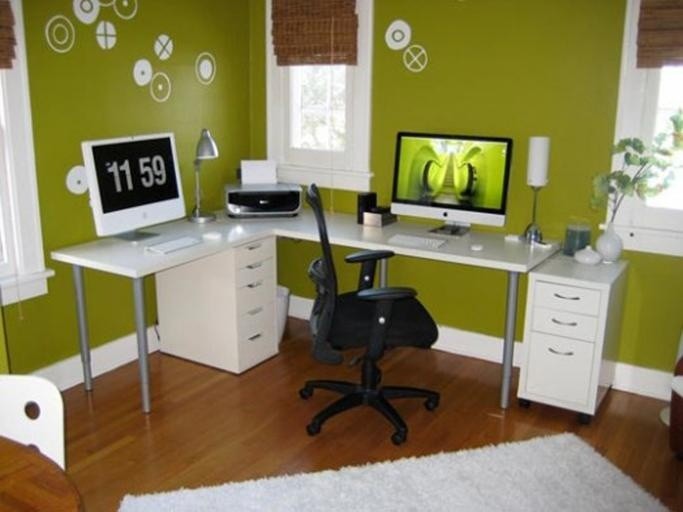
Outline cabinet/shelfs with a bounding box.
[516,252,630,425]
[155,235,279,375]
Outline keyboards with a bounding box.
[388,235,446,249]
[148,237,198,253]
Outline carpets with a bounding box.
[118,432,672,512]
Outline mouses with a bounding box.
[469,242,484,251]
[203,230,222,241]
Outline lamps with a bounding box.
[191,129,219,224]
[523,138,549,244]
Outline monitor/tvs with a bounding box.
[388,131,514,238]
[82,132,188,246]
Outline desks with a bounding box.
[0,436,84,512]
[50,207,564,413]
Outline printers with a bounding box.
[225,181,302,218]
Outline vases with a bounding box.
[596,222,622,263]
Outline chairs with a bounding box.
[0,374,66,474]
[299,184,440,445]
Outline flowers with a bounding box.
[590,107,683,221]
